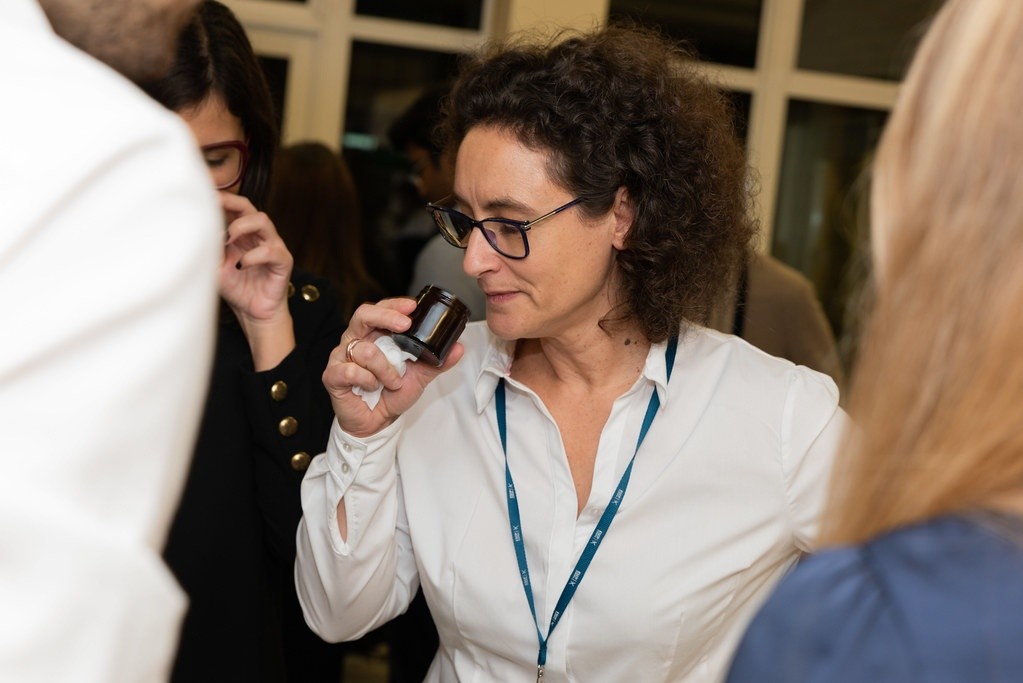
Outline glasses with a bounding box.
[425,192,586,259]
[198,136,249,190]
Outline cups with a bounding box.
[392,285,472,368]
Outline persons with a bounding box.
[0,0,1023,682]
